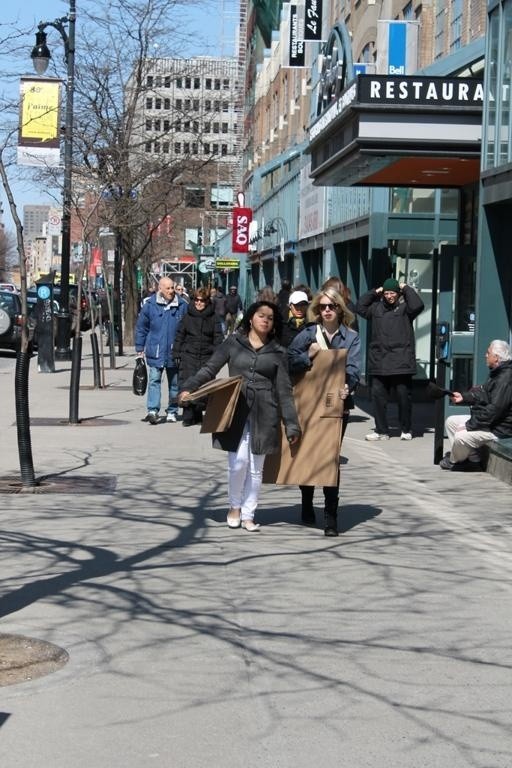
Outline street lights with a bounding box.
[31,14,72,363]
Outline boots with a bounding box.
[299,487,316,526]
[324,497,339,537]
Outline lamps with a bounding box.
[248,213,290,253]
[246,51,328,175]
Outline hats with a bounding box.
[383,278,401,293]
[427,382,455,398]
[289,290,309,305]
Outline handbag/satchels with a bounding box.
[133,356,147,396]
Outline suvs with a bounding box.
[0,291,23,357]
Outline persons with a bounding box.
[171,287,225,427]
[439,339,512,470]
[286,286,361,537]
[178,300,302,532]
[134,276,189,424]
[256,276,360,349]
[355,278,425,441]
[134,284,243,336]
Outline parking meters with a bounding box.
[432,318,452,467]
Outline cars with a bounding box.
[24,281,108,356]
[0,283,18,294]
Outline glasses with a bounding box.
[318,303,338,311]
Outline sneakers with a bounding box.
[241,521,260,532]
[366,432,389,441]
[226,510,241,527]
[400,430,413,440]
[148,410,158,424]
[166,413,176,422]
[439,456,462,469]
